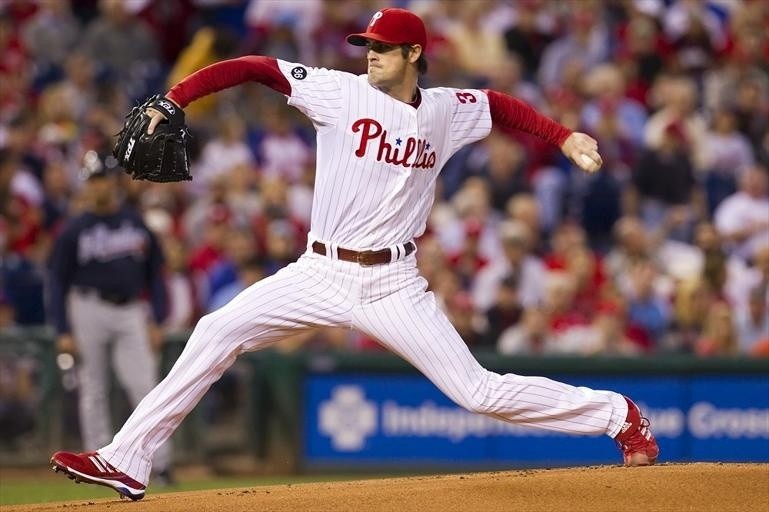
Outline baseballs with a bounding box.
[579,152,604,172]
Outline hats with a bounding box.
[346,9,426,49]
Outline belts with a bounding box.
[313,240,412,264]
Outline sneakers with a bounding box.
[48,451,145,501]
[614,396,658,465]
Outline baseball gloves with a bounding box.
[112,95,194,181]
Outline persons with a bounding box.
[2,0,768,360]
[50,8,659,502]
[47,149,171,496]
